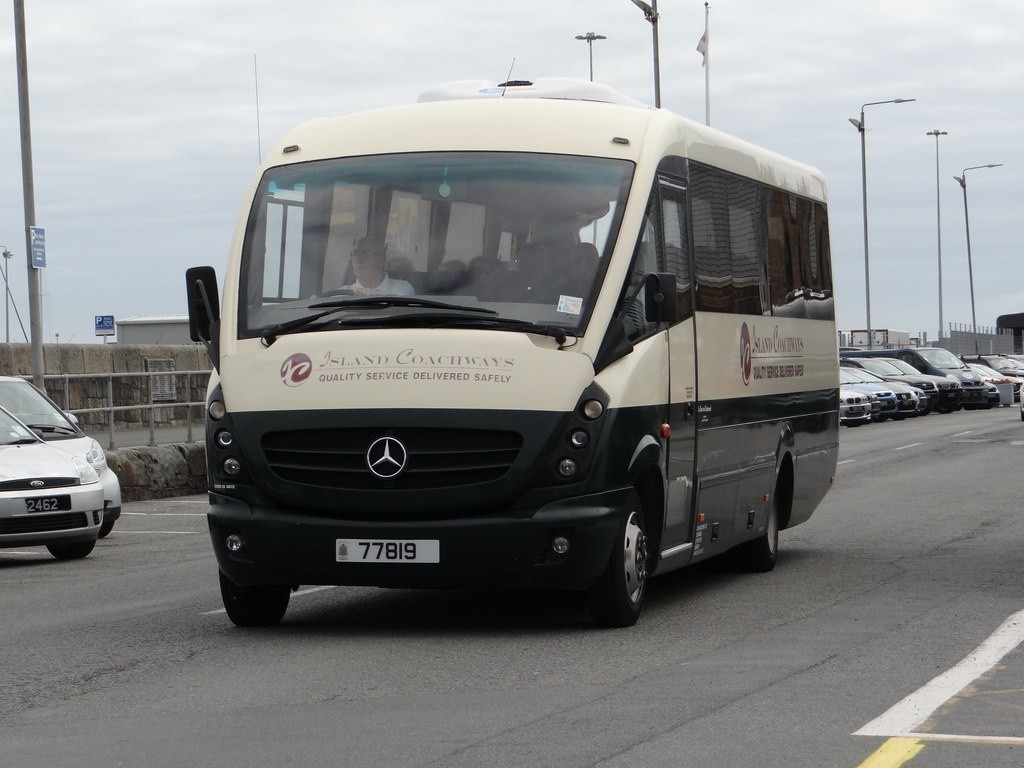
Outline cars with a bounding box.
[839,347,1024,427]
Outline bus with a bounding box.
[186,80,840,629]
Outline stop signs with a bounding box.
[29,226,47,268]
[95,314,115,336]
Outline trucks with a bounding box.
[851,329,920,348]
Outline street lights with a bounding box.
[575,32,607,81]
[631,0,661,109]
[849,98,916,348]
[953,164,1005,354]
[927,129,948,338]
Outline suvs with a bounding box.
[0,376,122,559]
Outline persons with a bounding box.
[309,231,600,305]
